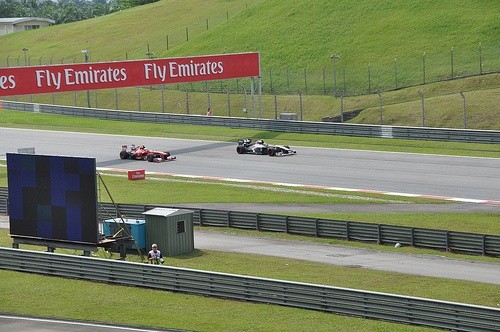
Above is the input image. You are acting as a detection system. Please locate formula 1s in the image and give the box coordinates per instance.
[236,139,296,156]
[119,145,176,163]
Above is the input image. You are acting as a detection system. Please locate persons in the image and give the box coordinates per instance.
[140,145,146,151]
[257,139,265,146]
[147,244,164,265]
[206,108,212,116]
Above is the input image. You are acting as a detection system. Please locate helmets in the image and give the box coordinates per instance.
[140,145,145,149]
[259,139,264,144]
